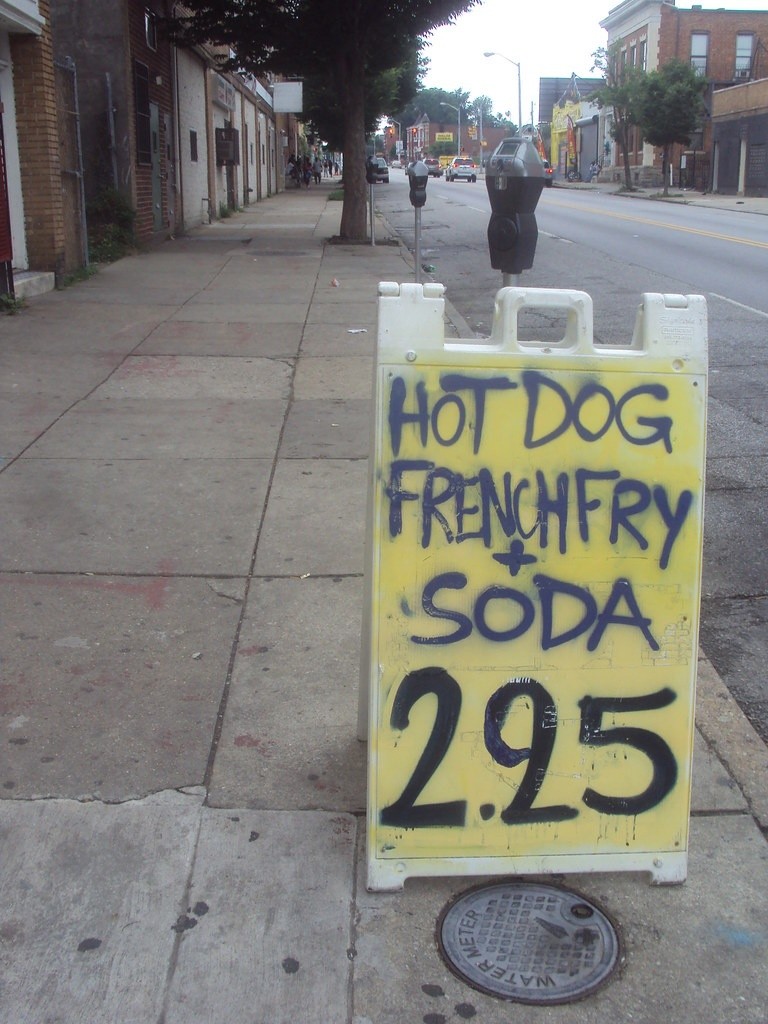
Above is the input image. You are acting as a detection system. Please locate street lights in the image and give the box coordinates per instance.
[387,119,402,161]
[467,101,483,175]
[439,102,461,157]
[483,51,523,136]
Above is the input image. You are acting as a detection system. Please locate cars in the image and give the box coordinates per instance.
[391,160,403,169]
[404,162,414,174]
[543,159,553,188]
[376,157,390,183]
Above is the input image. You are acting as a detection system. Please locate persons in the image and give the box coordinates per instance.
[323,160,339,177]
[586,161,601,182]
[286,154,323,190]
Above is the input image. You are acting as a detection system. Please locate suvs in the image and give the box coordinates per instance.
[422,158,443,177]
[444,157,477,182]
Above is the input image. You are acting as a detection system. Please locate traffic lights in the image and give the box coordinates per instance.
[467,127,473,137]
[472,126,476,135]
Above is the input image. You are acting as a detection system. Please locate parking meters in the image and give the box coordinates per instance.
[365,155,379,246]
[480,136,548,287]
[406,158,431,283]
